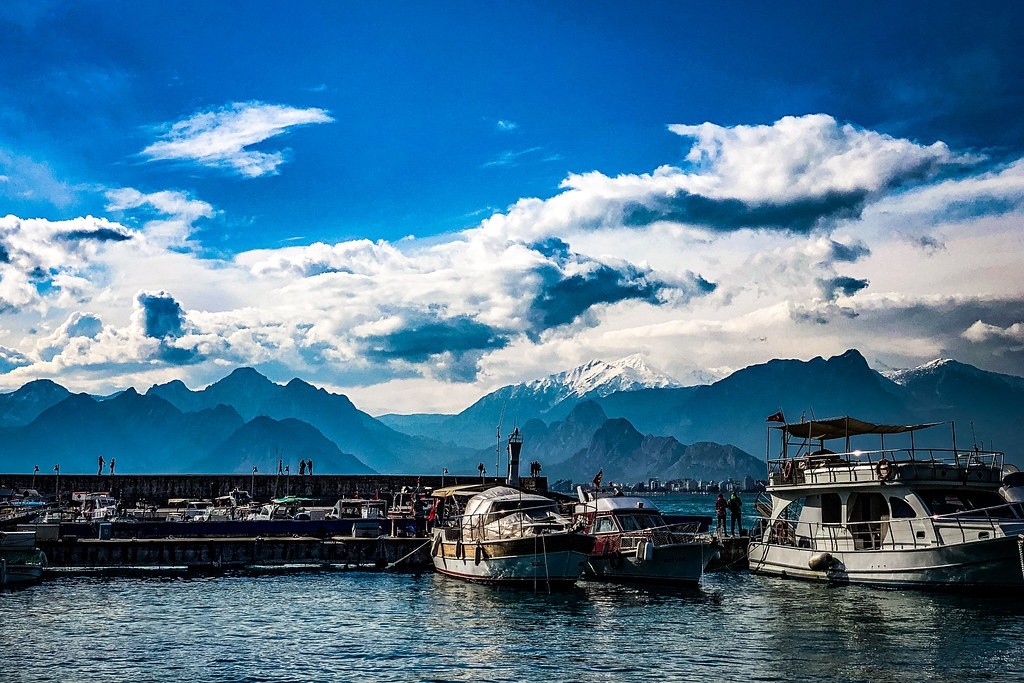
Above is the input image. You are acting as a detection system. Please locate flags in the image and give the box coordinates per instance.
[593,468,603,488]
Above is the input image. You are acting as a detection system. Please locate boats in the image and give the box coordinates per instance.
[0,559,42,589]
[747,405,1024,590]
[0,488,439,522]
[385,427,597,594]
[572,467,720,596]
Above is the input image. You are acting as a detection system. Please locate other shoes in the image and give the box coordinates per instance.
[730,535,735,539]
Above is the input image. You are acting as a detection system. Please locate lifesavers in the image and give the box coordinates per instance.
[784,462,792,480]
[609,550,623,570]
[876,459,893,481]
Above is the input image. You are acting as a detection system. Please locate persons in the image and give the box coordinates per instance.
[411,493,427,538]
[15,552,28,564]
[109,457,116,476]
[433,496,444,528]
[31,547,48,568]
[307,459,313,475]
[727,491,744,538]
[278,459,284,475]
[798,451,810,482]
[97,455,105,475]
[299,459,306,475]
[716,493,730,537]
